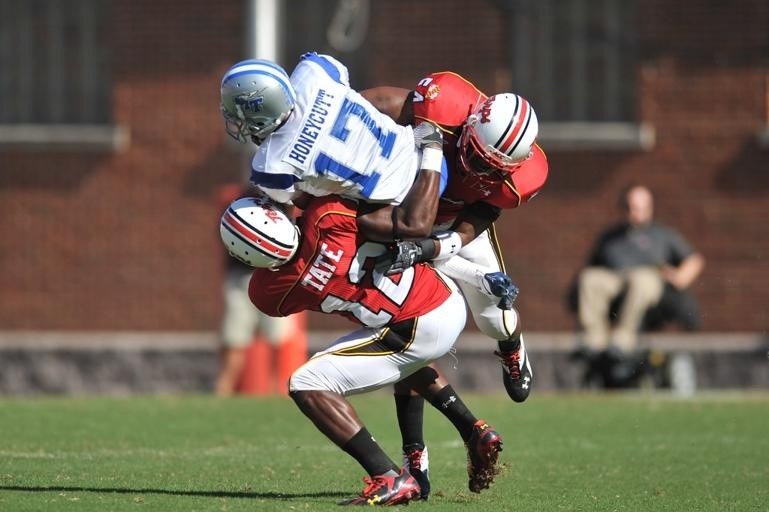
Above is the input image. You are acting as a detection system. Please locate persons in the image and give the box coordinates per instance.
[211,51,548,504]
[565,185,707,363]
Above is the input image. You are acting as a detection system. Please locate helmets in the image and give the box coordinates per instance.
[461,93,539,197]
[220,197,301,272]
[219,58,296,146]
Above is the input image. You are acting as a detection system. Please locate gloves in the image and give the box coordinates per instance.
[386,239,422,274]
[414,122,443,151]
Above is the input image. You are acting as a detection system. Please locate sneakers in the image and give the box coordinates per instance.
[339,467,421,506]
[464,419,503,493]
[494,333,533,402]
[476,268,518,309]
[403,442,430,498]
